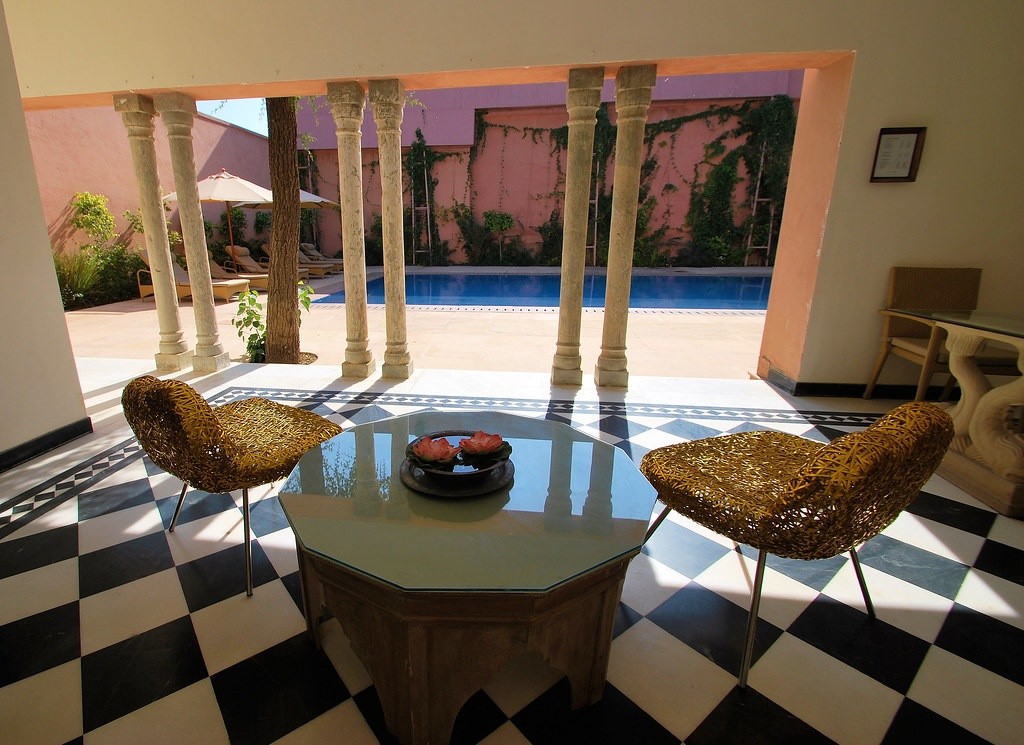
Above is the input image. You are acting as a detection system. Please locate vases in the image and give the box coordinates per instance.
[406,428,508,490]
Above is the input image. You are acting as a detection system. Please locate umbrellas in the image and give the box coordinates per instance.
[233,188,340,208]
[163,168,272,273]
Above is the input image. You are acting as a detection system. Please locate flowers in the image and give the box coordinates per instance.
[405,430,513,472]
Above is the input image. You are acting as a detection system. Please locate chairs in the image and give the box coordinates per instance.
[136,243,343,304]
[120,373,344,597]
[863,267,1024,403]
[640,402,957,707]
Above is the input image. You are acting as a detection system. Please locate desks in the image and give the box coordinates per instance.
[876,308,1024,520]
[278,409,660,745]
